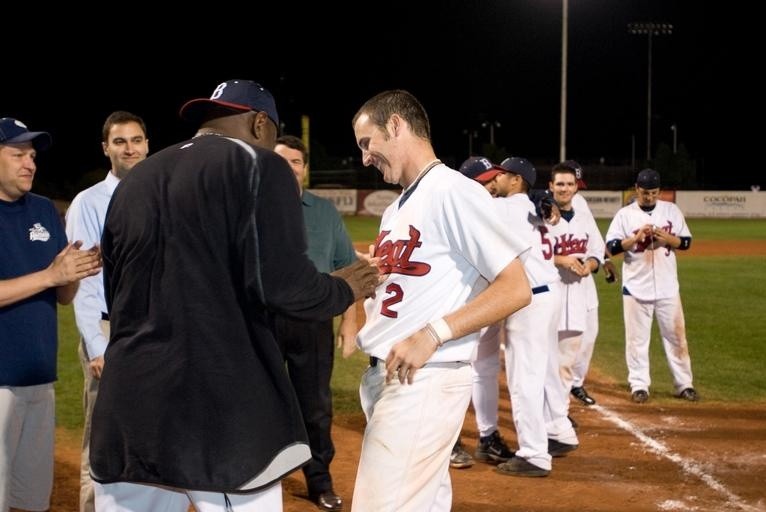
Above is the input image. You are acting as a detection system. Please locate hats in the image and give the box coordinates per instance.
[493,158,538,185]
[179,79,282,130]
[461,156,500,184]
[0,117,51,145]
[637,169,662,190]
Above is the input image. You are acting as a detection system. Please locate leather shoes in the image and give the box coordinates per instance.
[316,491,343,509]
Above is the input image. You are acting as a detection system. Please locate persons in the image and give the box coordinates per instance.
[90,82,379,512]
[351,90,532,512]
[275,136,355,512]
[0,116,104,510]
[65,112,151,512]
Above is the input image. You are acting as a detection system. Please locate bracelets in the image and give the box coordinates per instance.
[428,312,453,346]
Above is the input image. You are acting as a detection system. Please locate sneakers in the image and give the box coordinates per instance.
[496,454,552,478]
[633,389,648,404]
[675,387,698,401]
[549,436,580,457]
[449,444,475,469]
[569,385,598,406]
[476,437,514,464]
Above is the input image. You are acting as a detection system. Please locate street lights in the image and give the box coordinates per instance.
[667,123,679,157]
[623,21,673,159]
[482,114,505,151]
[461,126,478,154]
